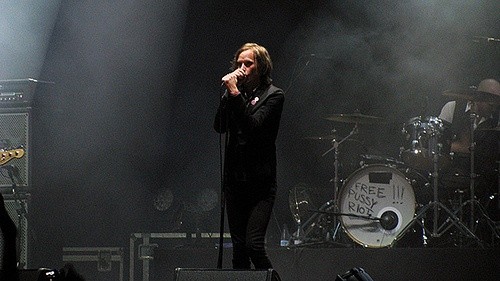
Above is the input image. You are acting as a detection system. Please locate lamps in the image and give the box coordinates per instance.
[153,186,178,212]
[196,188,220,212]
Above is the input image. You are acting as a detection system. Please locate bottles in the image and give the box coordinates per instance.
[279,224,291,247]
[293,224,305,245]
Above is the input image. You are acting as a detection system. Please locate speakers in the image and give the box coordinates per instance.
[0,106,51,271]
[174,268,281,281]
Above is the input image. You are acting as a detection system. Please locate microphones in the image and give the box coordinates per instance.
[471,36,500,46]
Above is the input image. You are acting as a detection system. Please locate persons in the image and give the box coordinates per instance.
[438,79,500,192]
[213,43,285,269]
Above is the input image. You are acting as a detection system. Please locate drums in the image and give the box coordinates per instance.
[336,165,432,249]
[355,153,404,169]
[399,115,456,172]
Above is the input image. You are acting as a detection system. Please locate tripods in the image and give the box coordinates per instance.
[290,141,348,244]
[390,103,500,247]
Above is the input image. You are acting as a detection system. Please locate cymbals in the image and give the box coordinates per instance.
[473,123,500,136]
[439,85,500,103]
[302,133,359,144]
[321,111,385,126]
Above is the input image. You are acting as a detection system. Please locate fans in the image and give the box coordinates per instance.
[288,183,314,246]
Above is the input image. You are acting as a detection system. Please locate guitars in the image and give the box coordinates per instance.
[0,144,26,167]
